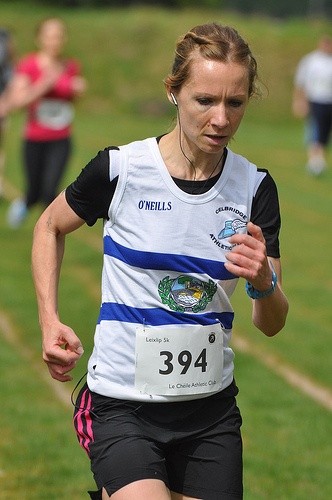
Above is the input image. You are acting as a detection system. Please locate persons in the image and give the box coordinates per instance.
[294,38,332,177]
[31,23,291,500]
[0,18,83,231]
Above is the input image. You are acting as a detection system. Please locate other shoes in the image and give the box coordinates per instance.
[10,200,27,226]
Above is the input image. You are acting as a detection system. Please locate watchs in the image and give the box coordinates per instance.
[244,267,277,299]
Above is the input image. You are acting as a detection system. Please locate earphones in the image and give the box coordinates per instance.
[170,92,178,107]
